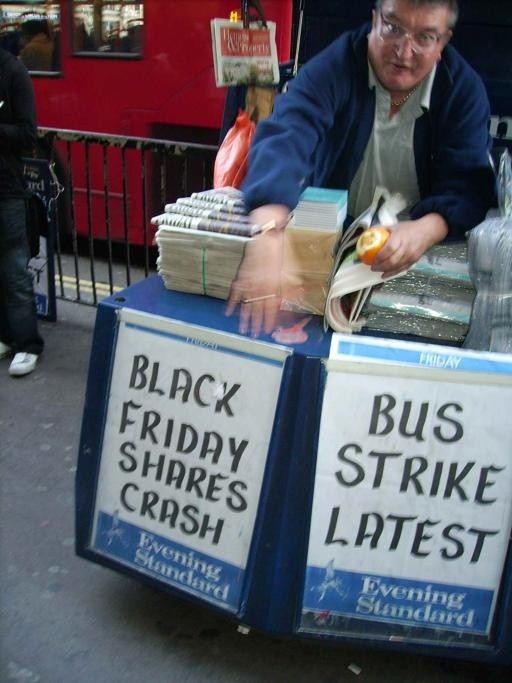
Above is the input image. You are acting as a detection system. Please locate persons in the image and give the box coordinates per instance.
[16,21,53,70]
[223,0,498,337]
[0,47,46,377]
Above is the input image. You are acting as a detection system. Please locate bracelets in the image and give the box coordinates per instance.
[249,218,287,238]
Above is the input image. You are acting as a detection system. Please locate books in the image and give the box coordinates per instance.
[322,183,477,346]
[148,185,349,315]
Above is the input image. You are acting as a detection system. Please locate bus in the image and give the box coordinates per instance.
[1,0,292,247]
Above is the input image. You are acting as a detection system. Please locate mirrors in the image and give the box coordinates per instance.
[0,0,64,76]
[67,2,143,59]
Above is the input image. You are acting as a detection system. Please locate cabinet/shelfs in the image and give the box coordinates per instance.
[75,275,512,669]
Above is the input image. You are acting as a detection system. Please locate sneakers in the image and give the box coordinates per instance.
[1,338,12,360]
[7,351,40,377]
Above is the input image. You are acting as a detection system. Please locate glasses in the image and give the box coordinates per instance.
[379,6,448,48]
